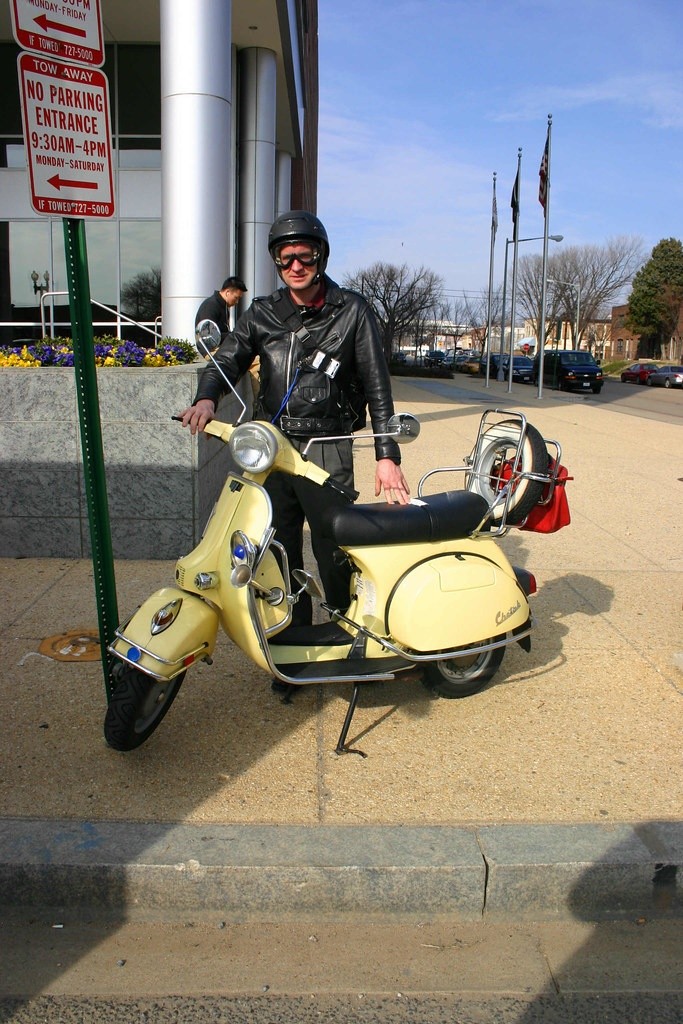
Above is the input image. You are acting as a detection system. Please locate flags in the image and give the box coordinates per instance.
[511,164,520,242]
[492,182,497,244]
[539,127,551,218]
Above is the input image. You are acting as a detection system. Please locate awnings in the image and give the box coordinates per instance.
[518,337,536,347]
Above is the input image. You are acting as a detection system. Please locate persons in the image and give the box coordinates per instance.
[195,276,260,405]
[178,209,410,694]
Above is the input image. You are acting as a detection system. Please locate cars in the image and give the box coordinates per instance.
[424,349,534,385]
[647,365,683,388]
[533,349,605,394]
[621,363,659,385]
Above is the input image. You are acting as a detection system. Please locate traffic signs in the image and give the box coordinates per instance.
[9,0,106,68]
[16,51,116,219]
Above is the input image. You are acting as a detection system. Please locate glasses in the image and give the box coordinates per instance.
[272,240,319,268]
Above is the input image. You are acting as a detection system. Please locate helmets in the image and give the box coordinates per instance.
[267,210,330,274]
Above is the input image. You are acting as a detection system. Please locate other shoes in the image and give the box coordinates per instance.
[272,676,301,693]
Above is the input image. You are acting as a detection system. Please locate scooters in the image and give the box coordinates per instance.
[102,319,575,759]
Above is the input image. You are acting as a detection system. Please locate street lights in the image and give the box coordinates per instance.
[547,280,581,350]
[498,235,563,381]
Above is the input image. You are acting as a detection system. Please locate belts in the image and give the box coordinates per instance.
[253,413,354,433]
